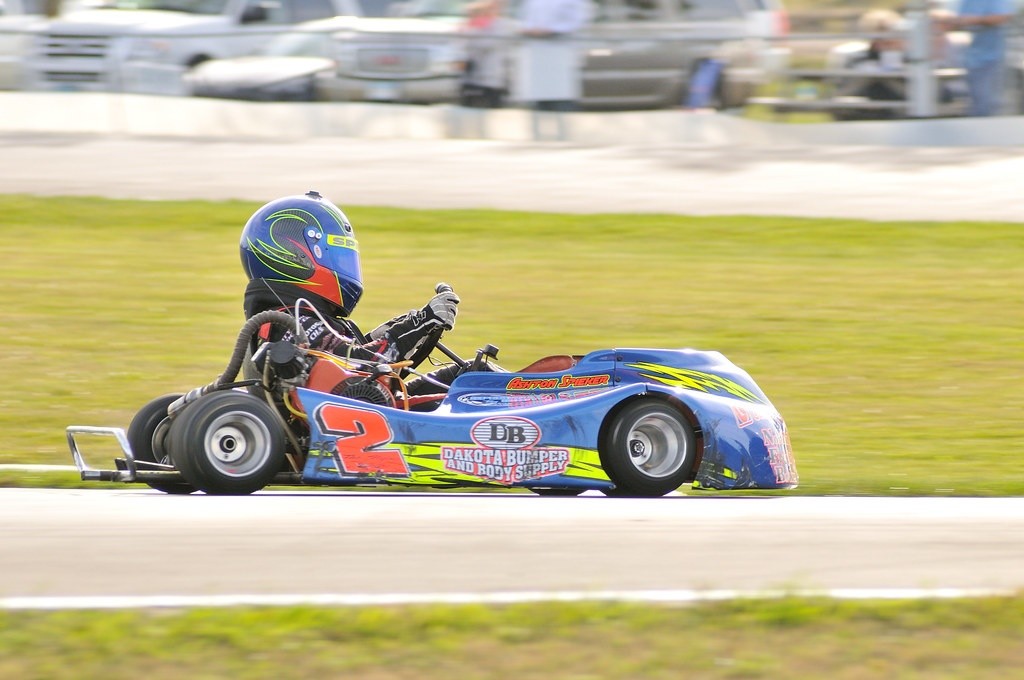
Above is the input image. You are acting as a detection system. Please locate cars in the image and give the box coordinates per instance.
[182,14,374,105]
[312,0,791,117]
[21,0,395,95]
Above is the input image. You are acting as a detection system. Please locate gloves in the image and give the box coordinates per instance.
[418,291,460,330]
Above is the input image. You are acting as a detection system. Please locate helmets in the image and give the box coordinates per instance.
[240,191,363,316]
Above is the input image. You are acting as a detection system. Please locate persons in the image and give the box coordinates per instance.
[239,191,512,412]
[934,0,1017,116]
[450,0,591,112]
[832,9,907,120]
